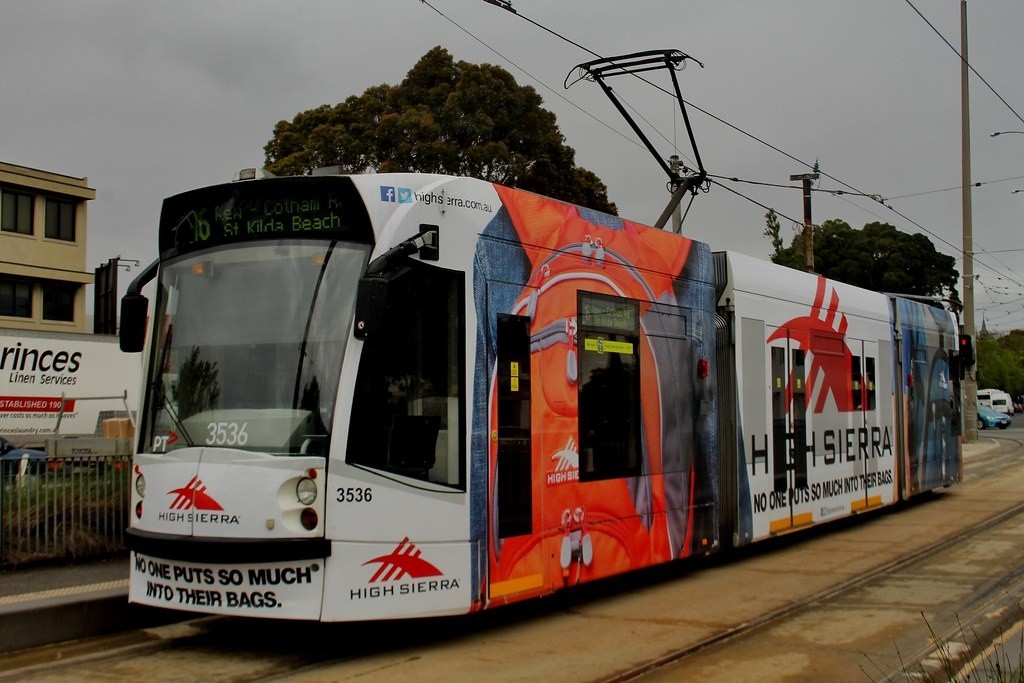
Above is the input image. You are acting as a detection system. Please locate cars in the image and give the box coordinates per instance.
[977,406,1011,430]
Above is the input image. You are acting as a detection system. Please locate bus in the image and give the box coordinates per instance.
[118,48,965,624]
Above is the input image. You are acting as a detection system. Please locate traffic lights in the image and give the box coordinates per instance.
[960,334,972,366]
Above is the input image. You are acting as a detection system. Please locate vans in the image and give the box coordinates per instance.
[977,388,1014,416]
